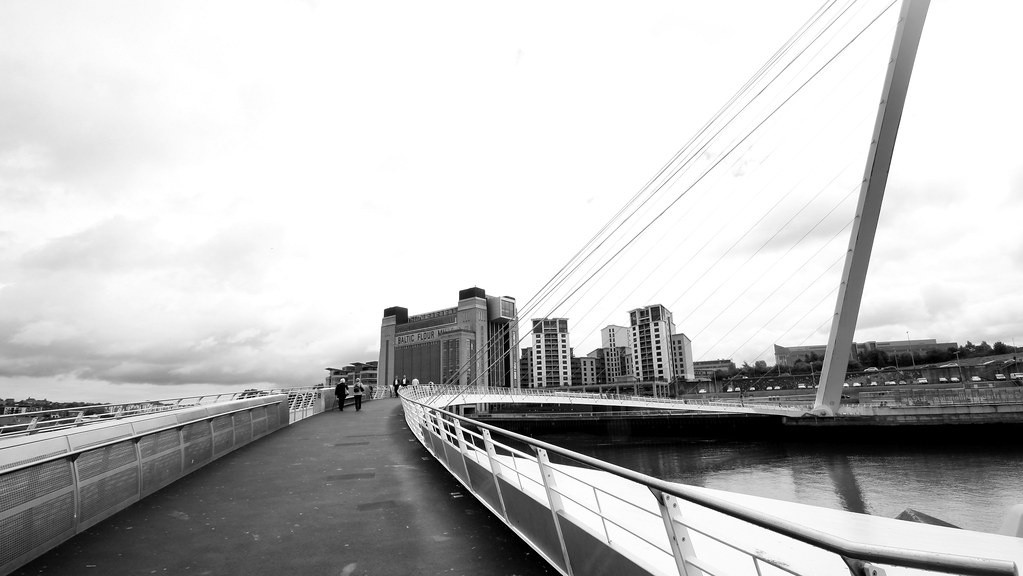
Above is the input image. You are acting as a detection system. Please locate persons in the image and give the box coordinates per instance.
[335,378,348,411]
[684,400,687,405]
[393,376,400,398]
[354,378,365,411]
[412,376,419,395]
[401,374,409,395]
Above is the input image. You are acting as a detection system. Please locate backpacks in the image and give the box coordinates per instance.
[354,384,359,392]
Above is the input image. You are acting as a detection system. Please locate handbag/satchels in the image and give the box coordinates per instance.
[360,383,364,392]
[345,388,350,395]
